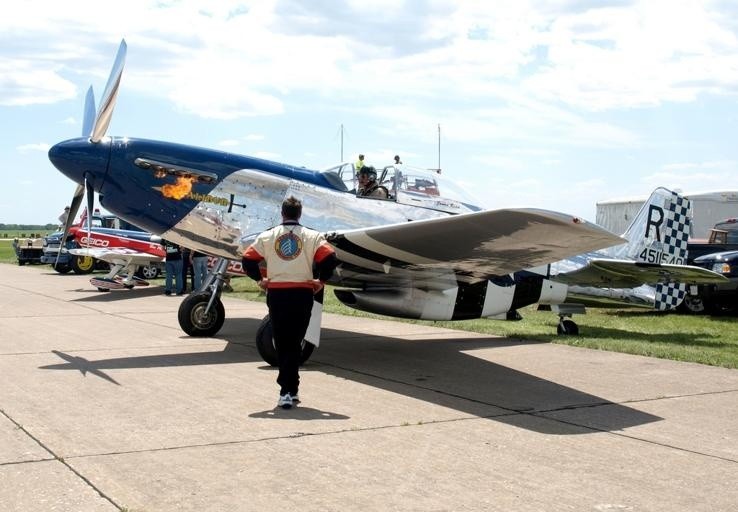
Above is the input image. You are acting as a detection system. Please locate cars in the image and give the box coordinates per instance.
[137,260,193,280]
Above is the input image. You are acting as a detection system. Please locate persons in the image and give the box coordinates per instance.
[357,166,388,199]
[59,207,69,225]
[356,155,365,179]
[242,197,337,409]
[160,238,208,295]
[394,156,402,164]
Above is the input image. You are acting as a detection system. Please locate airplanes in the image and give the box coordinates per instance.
[66,206,271,294]
[46,38,731,339]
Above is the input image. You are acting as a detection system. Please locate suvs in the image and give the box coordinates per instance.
[690,250,738,316]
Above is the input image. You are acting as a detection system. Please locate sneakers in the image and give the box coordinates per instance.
[278,393,299,409]
[166,291,171,295]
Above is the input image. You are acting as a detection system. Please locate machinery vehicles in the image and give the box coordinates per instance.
[677,217,737,314]
[39,209,154,274]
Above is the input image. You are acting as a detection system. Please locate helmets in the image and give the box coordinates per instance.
[360,166,377,182]
